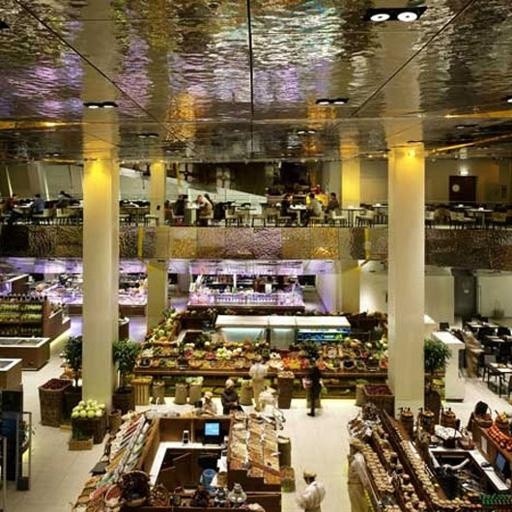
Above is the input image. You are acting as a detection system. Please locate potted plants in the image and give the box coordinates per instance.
[61,335,83,416]
[424,339,453,412]
[112,340,142,411]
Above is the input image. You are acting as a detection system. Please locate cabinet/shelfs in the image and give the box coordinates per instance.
[73,408,510,512]
[133,312,386,398]
[1,293,77,387]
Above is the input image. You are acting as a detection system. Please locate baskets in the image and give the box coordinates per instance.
[68,433,94,451]
[474,402,493,427]
[148,484,170,506]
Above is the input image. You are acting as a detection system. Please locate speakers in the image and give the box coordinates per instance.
[183,430,189,444]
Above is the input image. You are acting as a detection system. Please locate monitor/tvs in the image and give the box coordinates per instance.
[493,451,510,483]
[201,421,224,443]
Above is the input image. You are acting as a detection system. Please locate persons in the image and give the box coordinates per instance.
[2,193,18,222]
[324,192,339,224]
[302,193,322,227]
[201,391,217,413]
[166,194,215,225]
[280,193,296,228]
[54,194,80,209]
[293,470,325,511]
[28,192,46,225]
[211,324,226,343]
[307,364,325,417]
[220,377,244,414]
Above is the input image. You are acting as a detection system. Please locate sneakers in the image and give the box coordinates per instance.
[307,412,314,417]
[254,406,261,412]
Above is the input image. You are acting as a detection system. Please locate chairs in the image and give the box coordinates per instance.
[425,200,511,227]
[34,200,81,223]
[220,199,388,228]
[120,202,181,224]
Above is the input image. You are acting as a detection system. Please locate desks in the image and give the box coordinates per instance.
[441,313,512,400]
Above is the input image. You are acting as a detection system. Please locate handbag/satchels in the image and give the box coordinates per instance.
[230,405,245,413]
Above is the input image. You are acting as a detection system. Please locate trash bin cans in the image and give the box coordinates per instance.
[130,379,150,405]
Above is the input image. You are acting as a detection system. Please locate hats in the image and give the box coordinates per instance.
[303,467,318,478]
[347,436,363,450]
[225,379,234,389]
[204,391,213,400]
